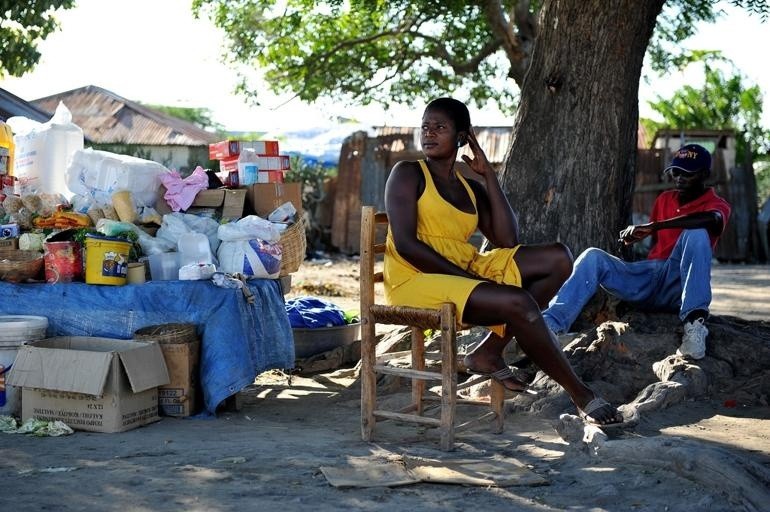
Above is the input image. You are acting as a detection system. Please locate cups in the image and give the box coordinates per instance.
[127,262,145,286]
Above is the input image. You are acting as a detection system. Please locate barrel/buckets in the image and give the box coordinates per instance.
[85,232,133,286]
[179,229,212,266]
[0,314,49,415]
[43,241,81,283]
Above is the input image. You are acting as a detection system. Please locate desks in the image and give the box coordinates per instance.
[0,280,296,420]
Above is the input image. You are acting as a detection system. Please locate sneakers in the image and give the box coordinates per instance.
[676,319,710,360]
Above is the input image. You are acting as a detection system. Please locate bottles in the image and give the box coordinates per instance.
[237,148,259,187]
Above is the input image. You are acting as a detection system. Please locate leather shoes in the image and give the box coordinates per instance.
[663,144,712,175]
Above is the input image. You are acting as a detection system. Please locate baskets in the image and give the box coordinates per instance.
[277,215,308,276]
[132,323,196,344]
[1,249,43,281]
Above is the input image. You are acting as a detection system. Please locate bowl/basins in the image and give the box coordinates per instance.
[292,322,362,359]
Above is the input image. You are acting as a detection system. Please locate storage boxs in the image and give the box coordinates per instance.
[4,335,170,434]
[158,340,201,418]
[156,141,303,221]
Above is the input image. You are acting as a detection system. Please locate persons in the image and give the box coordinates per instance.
[543,144,734,359]
[382,97,625,427]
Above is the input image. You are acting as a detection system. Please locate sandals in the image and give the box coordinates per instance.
[467,365,529,394]
[570,396,624,427]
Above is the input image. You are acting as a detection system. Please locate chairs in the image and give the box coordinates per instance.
[360,205,505,453]
